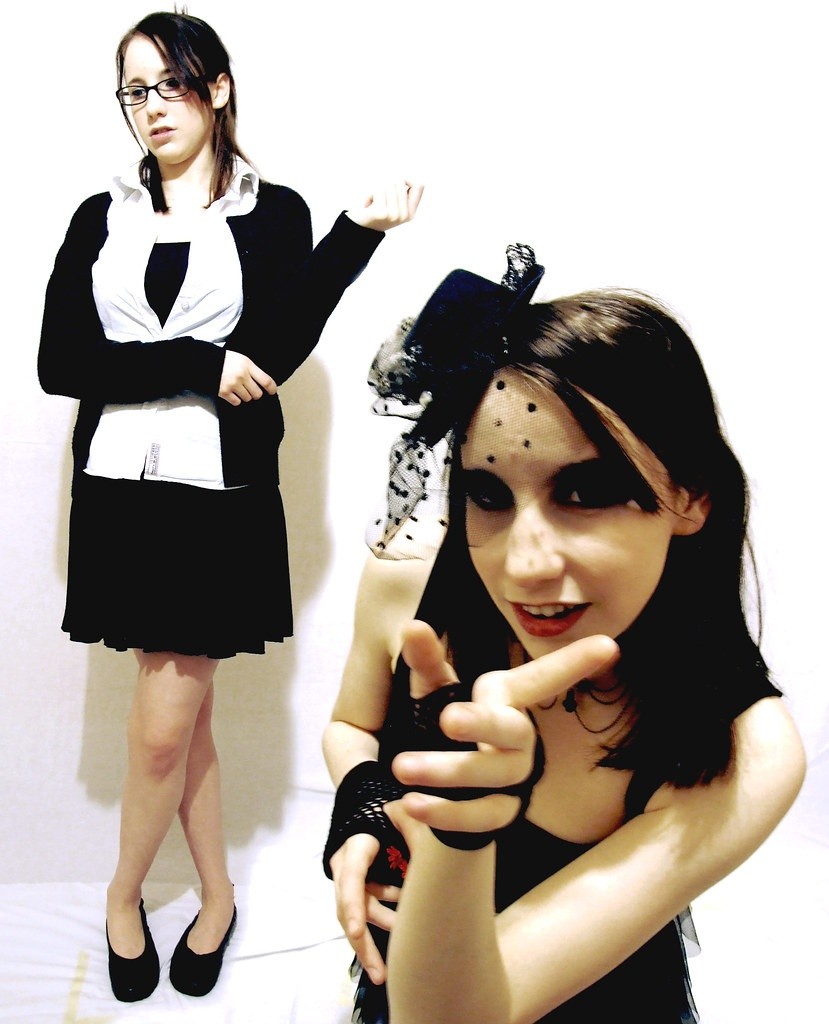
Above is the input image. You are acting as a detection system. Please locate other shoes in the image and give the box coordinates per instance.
[169,904,236,997]
[105,899,160,1003]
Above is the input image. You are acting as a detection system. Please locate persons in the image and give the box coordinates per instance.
[321,237,808,1024]
[35,11,428,1003]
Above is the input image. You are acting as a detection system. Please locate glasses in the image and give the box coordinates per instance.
[117,76,216,106]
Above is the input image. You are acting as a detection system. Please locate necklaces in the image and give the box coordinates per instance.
[562,678,634,734]
